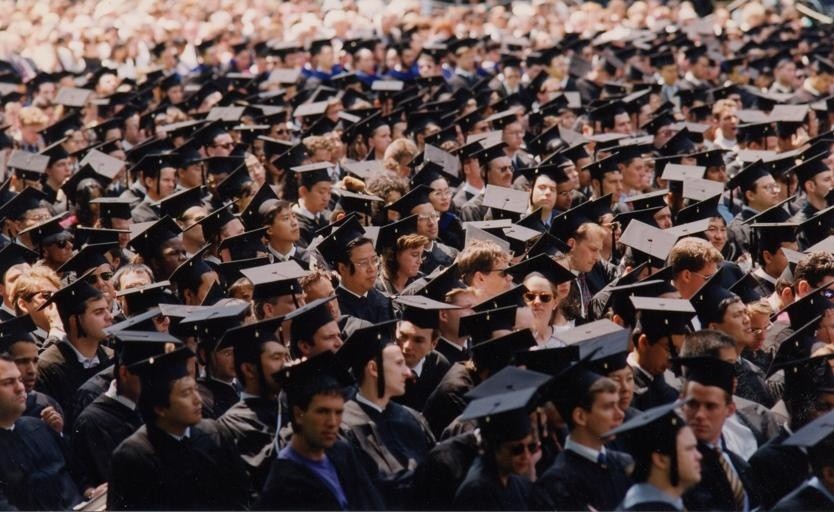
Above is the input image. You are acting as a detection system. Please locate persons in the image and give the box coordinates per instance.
[1,1,834,512]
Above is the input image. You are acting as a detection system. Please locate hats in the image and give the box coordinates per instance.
[0,315,38,341]
[208,106,246,122]
[404,112,436,136]
[539,346,602,403]
[668,355,738,393]
[255,112,290,125]
[172,140,200,156]
[104,92,130,105]
[517,206,546,234]
[738,109,769,140]
[273,142,314,171]
[424,142,460,177]
[294,102,326,123]
[683,175,724,205]
[385,183,434,215]
[604,279,663,315]
[425,101,454,114]
[56,243,113,275]
[635,135,654,154]
[552,201,594,240]
[213,261,260,285]
[464,364,552,399]
[234,125,265,140]
[376,215,417,254]
[6,148,47,180]
[651,156,681,176]
[491,93,521,112]
[423,127,456,144]
[677,192,722,222]
[291,162,332,181]
[78,150,125,178]
[239,261,308,299]
[782,408,834,468]
[604,84,623,95]
[128,347,195,382]
[160,303,204,332]
[503,223,542,257]
[39,115,80,138]
[511,346,580,375]
[502,54,522,67]
[338,190,381,212]
[104,310,162,346]
[258,136,293,157]
[60,169,100,198]
[630,296,696,335]
[359,111,388,128]
[272,349,346,394]
[417,263,462,299]
[456,109,481,131]
[116,281,172,314]
[521,233,569,257]
[623,88,652,111]
[186,202,234,236]
[397,95,423,111]
[673,86,706,105]
[685,44,707,63]
[503,252,575,286]
[765,314,833,395]
[529,123,560,155]
[89,198,140,219]
[449,138,486,155]
[40,137,69,161]
[482,184,530,220]
[810,96,833,119]
[458,387,539,440]
[624,190,668,210]
[337,320,395,369]
[687,149,732,166]
[151,186,203,218]
[549,91,581,109]
[217,163,250,201]
[457,305,517,336]
[410,161,444,187]
[573,329,631,374]
[462,219,512,237]
[797,205,834,242]
[166,119,205,136]
[727,159,767,190]
[804,138,834,159]
[561,142,589,160]
[168,245,212,284]
[650,52,673,68]
[605,58,617,76]
[54,87,91,112]
[94,120,118,133]
[450,87,471,103]
[719,58,743,73]
[728,274,759,303]
[472,78,490,93]
[581,152,619,179]
[108,330,180,363]
[631,84,661,94]
[468,142,508,163]
[640,113,677,135]
[630,64,643,80]
[191,155,243,174]
[240,181,280,230]
[219,228,270,260]
[612,206,661,231]
[815,55,833,75]
[669,122,710,143]
[302,116,335,139]
[73,228,132,242]
[0,187,47,220]
[660,163,707,192]
[765,147,807,172]
[601,399,688,446]
[217,317,283,353]
[752,91,792,109]
[619,219,678,268]
[541,149,568,165]
[770,105,809,124]
[372,76,403,102]
[659,126,695,155]
[705,84,736,97]
[664,218,709,239]
[471,327,537,364]
[130,154,177,170]
[19,211,69,241]
[742,193,796,223]
[0,241,39,284]
[750,223,796,242]
[589,132,627,147]
[603,137,641,162]
[784,151,829,183]
[485,110,517,128]
[315,212,362,238]
[654,100,675,115]
[589,101,628,127]
[394,295,459,328]
[35,268,98,313]
[180,303,248,341]
[316,215,365,265]
[769,282,833,331]
[691,266,742,321]
[124,137,169,156]
[125,220,183,254]
[689,106,712,119]
[593,193,613,215]
[471,284,528,312]
[284,296,337,337]
